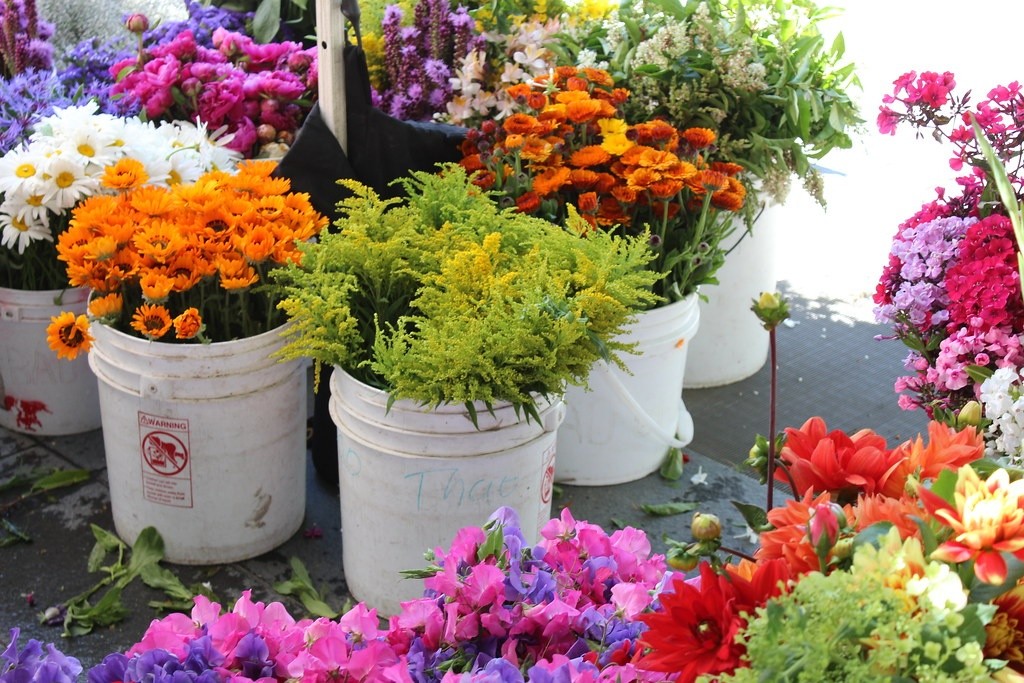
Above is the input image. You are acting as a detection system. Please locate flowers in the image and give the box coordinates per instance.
[0,0,1024,682]
